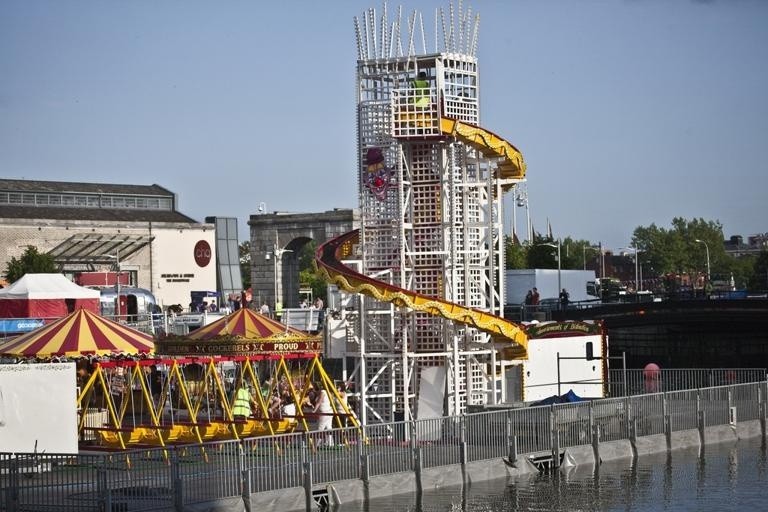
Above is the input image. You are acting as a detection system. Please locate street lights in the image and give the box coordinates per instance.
[696,239,712,281]
[534,236,642,306]
[99,247,123,319]
[265,229,294,311]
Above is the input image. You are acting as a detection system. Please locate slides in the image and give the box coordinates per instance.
[315,108,529,375]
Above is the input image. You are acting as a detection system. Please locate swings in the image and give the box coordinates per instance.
[94,359,303,446]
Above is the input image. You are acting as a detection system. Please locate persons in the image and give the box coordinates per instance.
[558,288,569,312]
[531,286,540,305]
[524,289,532,305]
[81,362,348,452]
[150,295,325,317]
